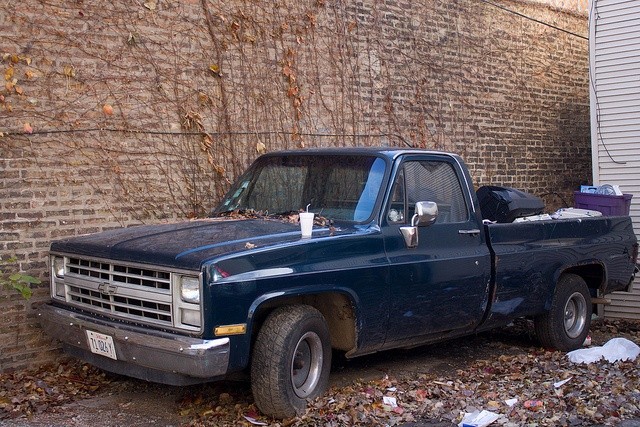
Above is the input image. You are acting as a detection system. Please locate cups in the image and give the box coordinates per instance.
[298,213,315,237]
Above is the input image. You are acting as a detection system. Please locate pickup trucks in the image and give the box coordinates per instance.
[36,146,639,419]
